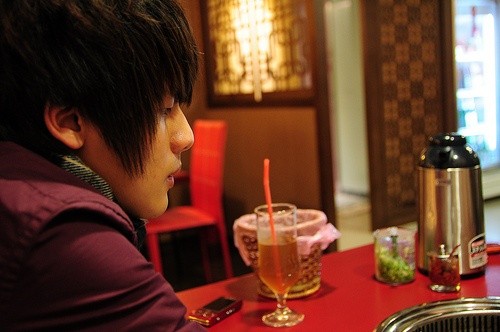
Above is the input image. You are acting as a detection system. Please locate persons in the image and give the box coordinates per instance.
[0,0,204,332]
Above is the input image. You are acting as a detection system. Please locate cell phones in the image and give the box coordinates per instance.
[186,297,242,325]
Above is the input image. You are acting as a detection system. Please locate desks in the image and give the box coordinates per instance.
[176,242,500,332]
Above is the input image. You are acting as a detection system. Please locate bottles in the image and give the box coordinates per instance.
[426,243,461,292]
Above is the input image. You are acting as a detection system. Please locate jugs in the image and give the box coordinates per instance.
[415,132,485,281]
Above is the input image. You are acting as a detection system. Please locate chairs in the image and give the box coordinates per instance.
[141,119,233,279]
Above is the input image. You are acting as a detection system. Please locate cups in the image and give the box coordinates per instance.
[372,226,416,285]
[238,209,328,299]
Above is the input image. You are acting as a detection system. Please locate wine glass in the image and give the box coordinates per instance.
[255,203,305,327]
